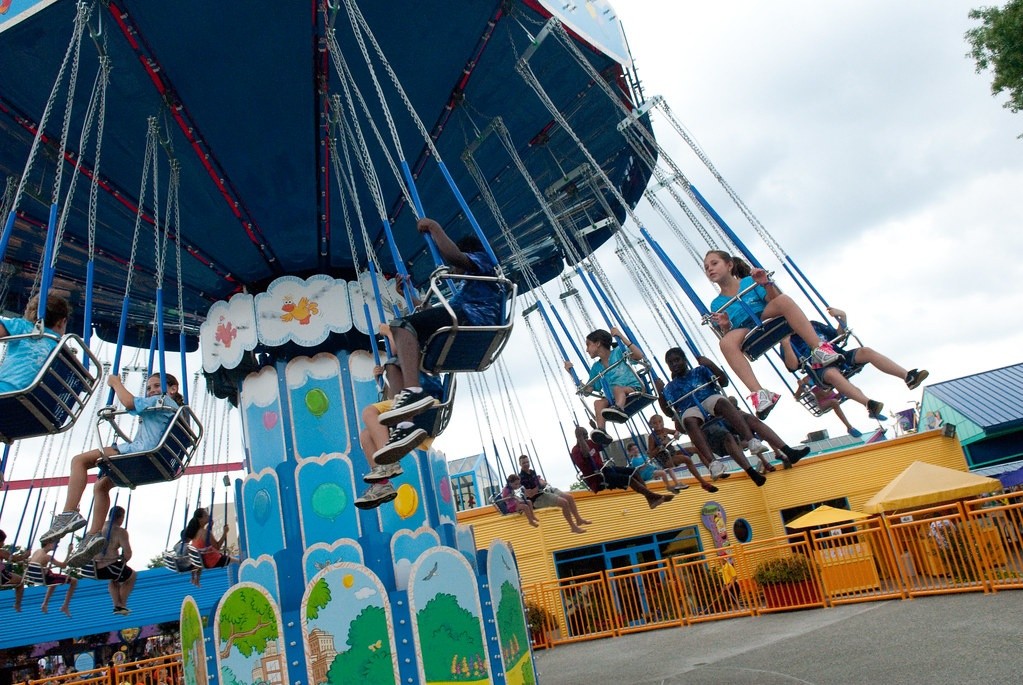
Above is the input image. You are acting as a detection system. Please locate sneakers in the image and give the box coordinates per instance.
[354,480,397,509]
[811,342,840,370]
[867,398,884,416]
[905,368,929,390]
[363,460,403,483]
[746,387,781,420]
[67,530,107,568]
[709,460,726,480]
[372,424,427,465]
[747,438,769,455]
[378,388,434,425]
[39,508,87,542]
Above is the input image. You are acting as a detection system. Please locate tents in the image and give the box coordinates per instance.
[864,460,1005,585]
[1000,466,1023,504]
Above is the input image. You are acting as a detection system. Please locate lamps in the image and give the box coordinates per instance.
[942,423,956,438]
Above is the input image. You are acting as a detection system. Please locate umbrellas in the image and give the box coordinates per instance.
[784,502,872,547]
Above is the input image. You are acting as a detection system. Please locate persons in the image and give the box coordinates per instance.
[24,638,184,685]
[40,374,184,567]
[354,218,503,510]
[184,508,238,568]
[648,415,730,492]
[519,455,592,533]
[502,474,539,529]
[94,506,135,615]
[30,538,77,618]
[565,327,646,444]
[572,419,693,509]
[0,290,68,487]
[173,529,200,588]
[704,251,847,421]
[655,348,811,488]
[780,307,930,437]
[920,512,956,547]
[0,529,32,612]
[721,559,739,611]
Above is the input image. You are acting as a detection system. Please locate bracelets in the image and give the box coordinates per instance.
[729,321,734,330]
[628,342,633,347]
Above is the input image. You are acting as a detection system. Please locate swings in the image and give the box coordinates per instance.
[92,99,206,491]
[320,0,522,442]
[452,3,869,518]
[0,332,232,589]
[0,3,114,442]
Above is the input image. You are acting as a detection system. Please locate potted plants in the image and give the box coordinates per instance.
[525,603,560,647]
[751,554,827,609]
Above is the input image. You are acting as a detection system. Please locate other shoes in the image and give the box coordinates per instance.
[113,606,132,614]
[600,405,628,423]
[591,429,613,445]
[868,412,888,421]
[647,447,809,511]
[847,427,862,437]
